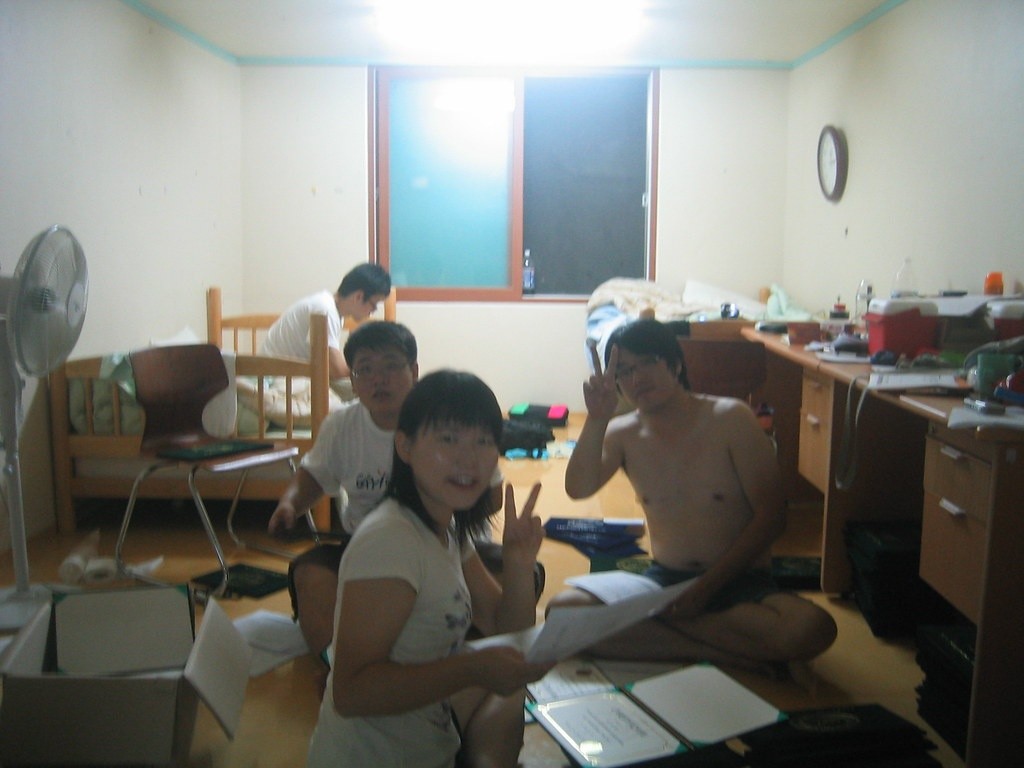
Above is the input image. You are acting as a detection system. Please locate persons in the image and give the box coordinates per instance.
[258,264,391,410]
[305,370,543,768]
[544,317,837,701]
[269,322,544,694]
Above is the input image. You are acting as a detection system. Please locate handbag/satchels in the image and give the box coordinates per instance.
[506,403,571,427]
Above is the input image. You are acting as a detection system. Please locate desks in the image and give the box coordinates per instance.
[740,327,1024,768]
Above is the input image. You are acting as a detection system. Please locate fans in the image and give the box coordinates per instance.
[0,224,90,630]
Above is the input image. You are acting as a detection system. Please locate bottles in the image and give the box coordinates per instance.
[523,249,535,292]
[897,255,916,291]
[856,278,869,318]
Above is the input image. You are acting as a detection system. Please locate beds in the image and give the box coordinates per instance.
[48,285,397,535]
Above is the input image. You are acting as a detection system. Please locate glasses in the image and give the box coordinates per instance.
[368,300,378,311]
[616,357,658,382]
[349,358,409,383]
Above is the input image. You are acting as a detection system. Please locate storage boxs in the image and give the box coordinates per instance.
[0,583,250,768]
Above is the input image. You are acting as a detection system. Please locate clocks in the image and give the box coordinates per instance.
[817,124,846,200]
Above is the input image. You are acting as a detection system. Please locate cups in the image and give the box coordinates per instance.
[977,353,1014,404]
[984,271,1003,295]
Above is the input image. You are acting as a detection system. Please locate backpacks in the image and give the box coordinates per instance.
[501,419,555,460]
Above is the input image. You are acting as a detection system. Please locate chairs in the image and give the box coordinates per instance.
[116,343,322,600]
[675,336,776,561]
